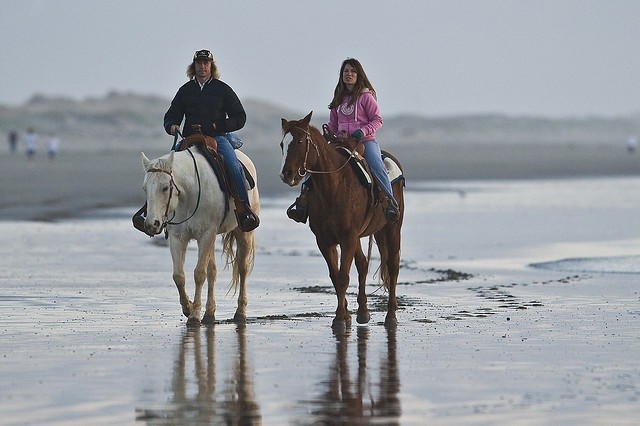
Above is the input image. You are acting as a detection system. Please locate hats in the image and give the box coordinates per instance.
[192,49,213,59]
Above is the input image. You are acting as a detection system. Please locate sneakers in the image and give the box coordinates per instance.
[385,206,397,219]
[239,213,256,230]
[133,215,155,237]
[289,206,306,223]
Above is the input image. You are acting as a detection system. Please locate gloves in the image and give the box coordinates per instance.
[324,132,333,141]
[353,128,363,140]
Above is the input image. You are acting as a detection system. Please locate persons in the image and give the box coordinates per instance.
[25,128,40,163]
[45,133,60,170]
[132,49,260,238]
[287,58,401,224]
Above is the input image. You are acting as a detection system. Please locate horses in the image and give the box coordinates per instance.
[139,143,261,327]
[278,110,405,335]
